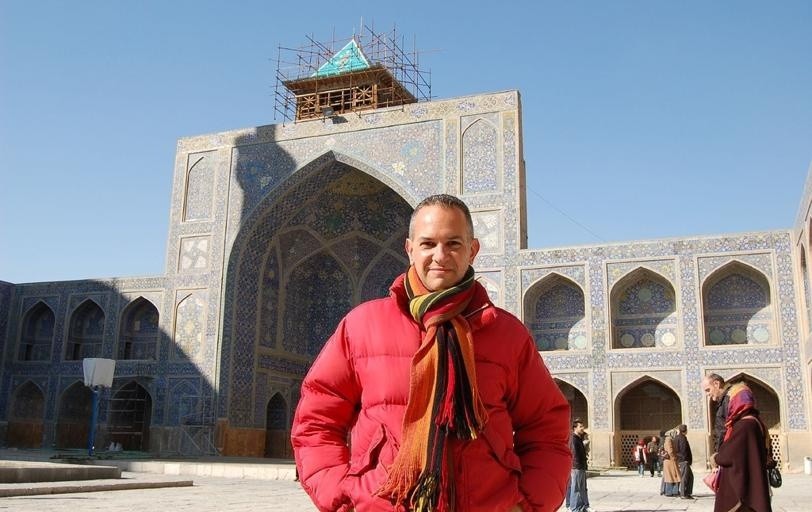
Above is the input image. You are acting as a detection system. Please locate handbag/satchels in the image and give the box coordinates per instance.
[770,468,782,488]
[657,447,671,460]
[703,468,719,491]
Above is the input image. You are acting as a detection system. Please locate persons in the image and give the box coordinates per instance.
[291,194,571,512]
[631,425,695,499]
[566,417,589,512]
[703,373,772,512]
